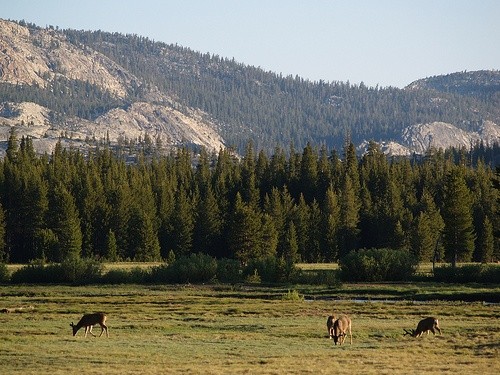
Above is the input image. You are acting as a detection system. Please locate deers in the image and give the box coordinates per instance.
[402,317,442,339]
[327,315,352,346]
[70,312,109,337]
